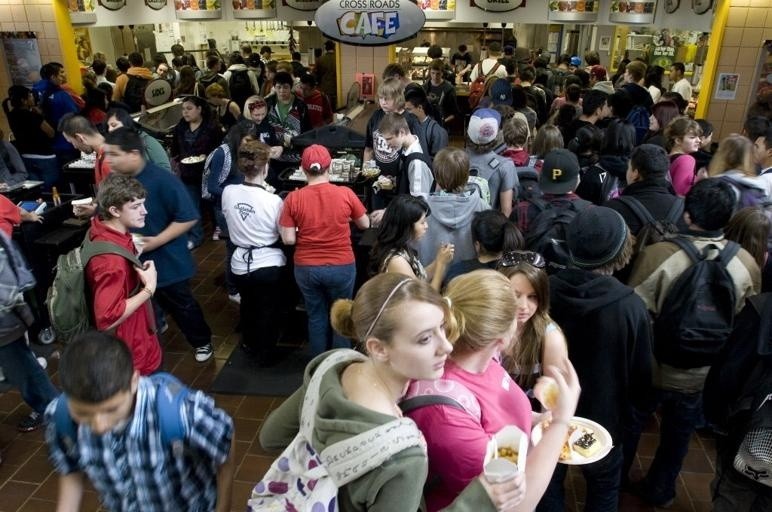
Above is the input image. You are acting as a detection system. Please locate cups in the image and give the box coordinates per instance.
[494,251,546,271]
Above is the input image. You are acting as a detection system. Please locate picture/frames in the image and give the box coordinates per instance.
[338,103,381,137]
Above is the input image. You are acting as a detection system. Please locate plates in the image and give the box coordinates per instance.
[410,61,430,79]
[610,26,697,76]
[14,191,89,320]
[273,166,374,212]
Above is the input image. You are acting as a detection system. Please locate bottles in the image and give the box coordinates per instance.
[301,143,331,172]
[625,61,647,77]
[466,108,501,145]
[538,147,580,195]
[584,50,600,66]
[504,45,513,55]
[570,56,581,65]
[488,41,501,51]
[490,78,514,107]
[262,46,275,54]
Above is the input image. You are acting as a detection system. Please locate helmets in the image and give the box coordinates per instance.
[180,156,204,165]
[531,417,613,466]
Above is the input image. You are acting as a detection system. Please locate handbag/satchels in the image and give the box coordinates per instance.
[143,287,153,298]
[547,415,572,428]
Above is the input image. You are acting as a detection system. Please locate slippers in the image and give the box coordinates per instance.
[247,348,428,512]
[468,61,501,109]
[551,70,571,97]
[721,175,771,212]
[565,206,627,270]
[613,194,686,257]
[46,226,143,352]
[617,87,650,142]
[31,84,65,124]
[228,69,252,104]
[524,198,593,277]
[0,231,37,313]
[514,156,541,202]
[201,143,232,199]
[461,155,513,204]
[717,292,772,496]
[124,73,153,115]
[656,236,741,371]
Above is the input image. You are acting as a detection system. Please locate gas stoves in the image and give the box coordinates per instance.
[379,175,392,190]
[71,196,94,217]
[572,431,598,459]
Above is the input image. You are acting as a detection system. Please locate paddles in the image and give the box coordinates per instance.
[210,339,307,396]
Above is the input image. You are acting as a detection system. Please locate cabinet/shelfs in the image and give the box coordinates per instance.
[52,186,61,207]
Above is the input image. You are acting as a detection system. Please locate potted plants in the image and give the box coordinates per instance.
[470,0,527,13]
[691,0,712,14]
[282,1,323,12]
[664,1,680,14]
[98,0,126,10]
[145,0,167,10]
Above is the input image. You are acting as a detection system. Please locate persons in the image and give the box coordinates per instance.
[113,56,130,84]
[504,63,517,82]
[377,113,437,198]
[2,84,61,193]
[574,69,590,98]
[404,86,449,161]
[382,63,435,116]
[753,130,772,197]
[549,205,655,512]
[500,117,544,173]
[449,44,472,79]
[206,39,226,73]
[223,51,260,114]
[222,95,269,146]
[291,51,304,77]
[259,60,278,99]
[404,145,493,275]
[42,331,235,512]
[739,114,770,143]
[511,84,538,132]
[544,53,576,81]
[532,122,565,159]
[603,143,689,288]
[108,109,172,173]
[92,60,117,90]
[156,62,170,77]
[0,130,29,189]
[0,194,45,239]
[644,100,679,147]
[597,118,636,188]
[622,50,631,64]
[669,62,692,116]
[517,66,547,128]
[723,206,772,268]
[755,87,772,107]
[553,103,577,148]
[150,53,180,89]
[83,52,117,83]
[596,93,634,134]
[570,90,610,139]
[748,101,771,122]
[612,60,654,118]
[643,65,664,104]
[440,210,525,296]
[198,55,231,98]
[259,71,313,184]
[98,84,133,114]
[172,55,188,72]
[569,56,581,73]
[295,74,334,129]
[241,44,265,87]
[533,57,553,87]
[221,140,297,367]
[567,124,620,206]
[541,51,552,71]
[81,87,110,130]
[663,116,704,195]
[427,86,441,105]
[172,45,200,73]
[398,268,581,512]
[610,59,631,86]
[478,73,499,101]
[276,61,295,75]
[549,74,583,115]
[707,133,757,215]
[422,59,461,130]
[257,272,524,512]
[709,251,772,512]
[564,84,584,120]
[366,194,456,294]
[111,52,155,114]
[493,104,513,129]
[490,77,531,138]
[172,65,205,98]
[427,44,452,81]
[469,40,507,81]
[621,178,763,506]
[589,65,615,94]
[0,227,61,432]
[694,119,715,174]
[205,82,242,131]
[49,62,88,112]
[186,54,204,81]
[313,41,336,110]
[500,45,518,66]
[32,63,81,161]
[533,73,553,112]
[57,112,112,220]
[508,148,594,251]
[462,107,520,218]
[260,45,275,64]
[278,143,370,360]
[362,76,432,211]
[83,172,163,375]
[657,92,688,114]
[693,36,708,65]
[583,49,601,74]
[104,127,213,362]
[496,249,569,512]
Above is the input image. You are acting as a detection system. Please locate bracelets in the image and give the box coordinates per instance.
[72,198,92,215]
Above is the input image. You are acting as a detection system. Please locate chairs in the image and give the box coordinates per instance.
[212,226,222,241]
[16,410,50,433]
[194,343,213,362]
[36,357,48,369]
[227,293,241,306]
